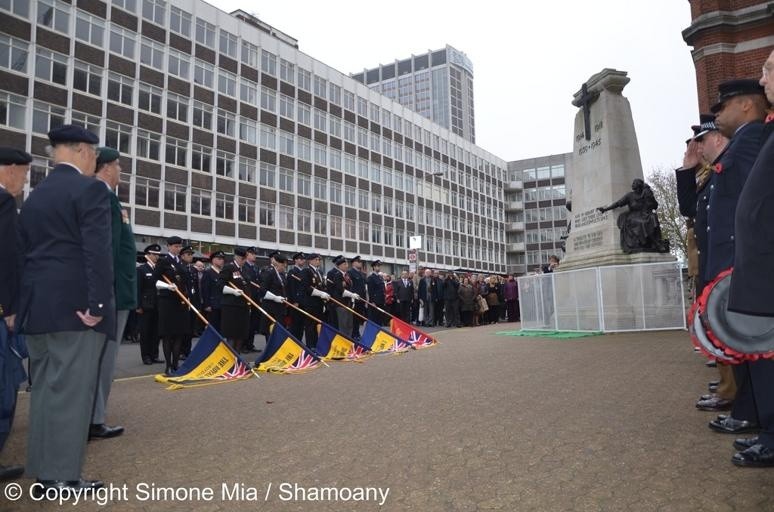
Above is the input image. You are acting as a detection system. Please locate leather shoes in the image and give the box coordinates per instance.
[0,462,24,480]
[251,347,261,352]
[696,380,774,466]
[88,424,123,440]
[241,348,249,353]
[144,353,192,376]
[34,475,103,499]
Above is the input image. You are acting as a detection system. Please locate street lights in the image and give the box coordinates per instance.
[412,172,443,274]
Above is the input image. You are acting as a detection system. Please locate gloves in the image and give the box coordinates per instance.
[310,288,330,304]
[222,285,244,297]
[154,279,177,292]
[342,289,360,302]
[263,290,286,304]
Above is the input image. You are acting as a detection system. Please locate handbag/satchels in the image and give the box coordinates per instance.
[477,295,489,312]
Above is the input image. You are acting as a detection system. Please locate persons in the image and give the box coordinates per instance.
[124,237,559,373]
[0,145,33,481]
[14,124,118,500]
[597,178,661,253]
[89,147,138,440]
[675,50,773,468]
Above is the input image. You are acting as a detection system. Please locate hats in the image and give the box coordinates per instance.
[144,236,195,256]
[332,255,361,266]
[47,125,99,144]
[710,79,765,113]
[95,147,119,164]
[209,247,257,259]
[371,260,382,266]
[694,114,718,141]
[0,146,32,166]
[268,249,320,263]
[691,126,700,133]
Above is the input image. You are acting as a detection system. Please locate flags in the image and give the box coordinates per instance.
[154,323,253,388]
